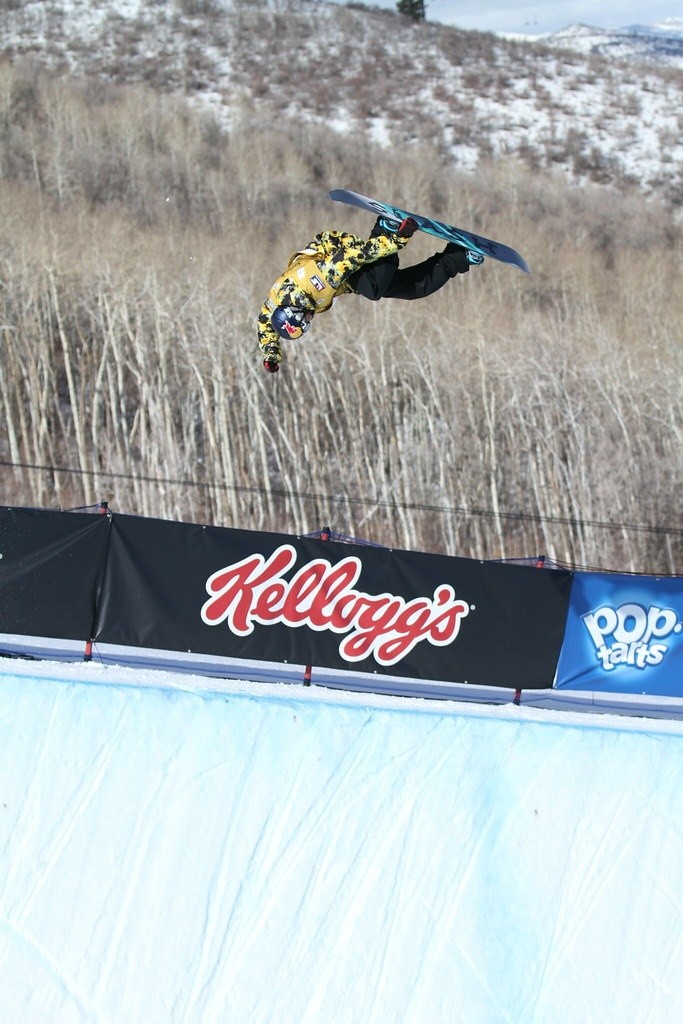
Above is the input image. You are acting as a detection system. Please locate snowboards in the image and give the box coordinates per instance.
[326,188,535,277]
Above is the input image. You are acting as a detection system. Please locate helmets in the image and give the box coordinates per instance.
[271,308,303,340]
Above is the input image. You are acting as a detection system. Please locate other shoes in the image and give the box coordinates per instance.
[381,218,401,232]
[466,250,484,266]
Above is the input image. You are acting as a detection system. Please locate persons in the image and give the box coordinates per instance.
[258,215,484,373]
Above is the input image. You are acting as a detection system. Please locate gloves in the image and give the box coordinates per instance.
[264,360,280,373]
[398,217,418,237]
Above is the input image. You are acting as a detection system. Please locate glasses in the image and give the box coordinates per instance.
[299,321,310,333]
